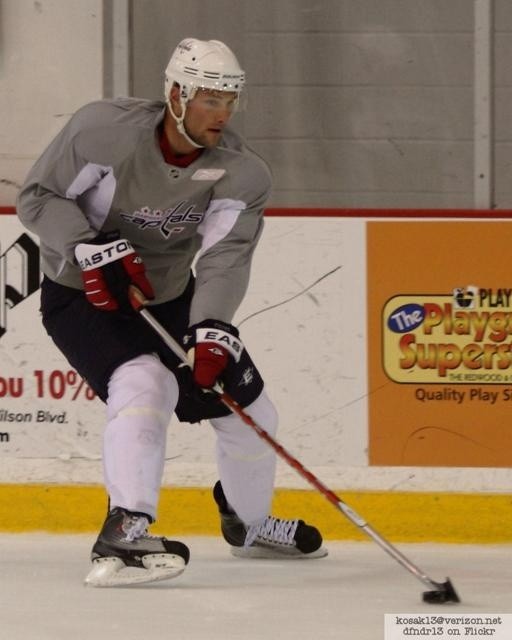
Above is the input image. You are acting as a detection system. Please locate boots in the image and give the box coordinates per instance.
[213,482,323,554]
[91,495,191,568]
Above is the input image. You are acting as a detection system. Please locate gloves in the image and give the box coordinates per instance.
[73,232,156,315]
[179,317,244,395]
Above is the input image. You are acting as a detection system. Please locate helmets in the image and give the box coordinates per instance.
[165,38,246,104]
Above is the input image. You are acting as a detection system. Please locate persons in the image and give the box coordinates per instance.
[15,36,323,569]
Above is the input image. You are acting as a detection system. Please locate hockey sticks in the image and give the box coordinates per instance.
[130,286,458,606]
